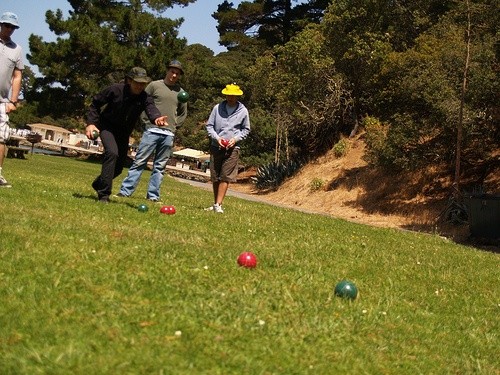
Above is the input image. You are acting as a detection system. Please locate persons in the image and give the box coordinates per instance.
[204,84,251,215]
[116,60,188,203]
[85,66,169,203]
[0,12,26,184]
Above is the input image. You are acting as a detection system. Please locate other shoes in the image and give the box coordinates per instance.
[98,195,110,203]
[0,175,12,188]
[154,198,163,204]
[112,192,129,198]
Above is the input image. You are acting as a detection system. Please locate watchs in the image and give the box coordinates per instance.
[10,101,18,107]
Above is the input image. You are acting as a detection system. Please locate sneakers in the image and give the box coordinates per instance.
[213,203,223,214]
[204,206,214,211]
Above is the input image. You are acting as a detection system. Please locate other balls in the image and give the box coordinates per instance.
[90,130,99,140]
[334,282,358,301]
[178,91,190,102]
[138,204,149,212]
[221,139,228,146]
[237,252,257,268]
[160,206,176,214]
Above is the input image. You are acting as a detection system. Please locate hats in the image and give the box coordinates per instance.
[0,12,20,29]
[222,84,243,96]
[166,60,185,76]
[126,66,151,83]
